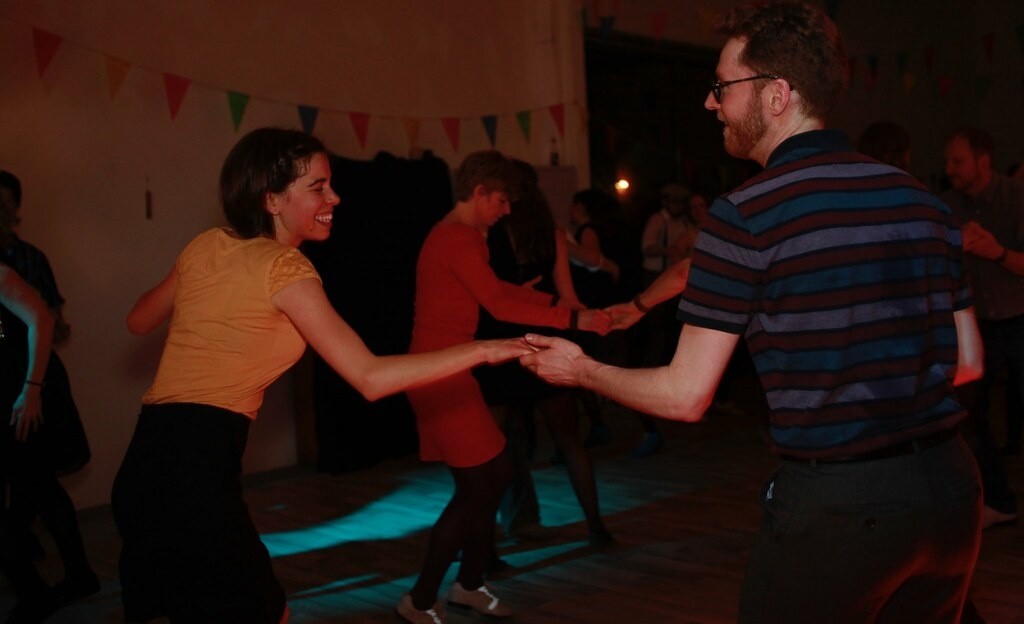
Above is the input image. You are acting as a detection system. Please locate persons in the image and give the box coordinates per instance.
[84,124,543,624]
[939,119,1024,529]
[859,119,990,255]
[0,167,89,623]
[519,6,986,624]
[375,148,714,624]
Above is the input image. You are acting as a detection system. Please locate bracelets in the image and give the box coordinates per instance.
[633,295,649,312]
[994,248,1008,264]
[570,308,579,330]
[550,296,560,307]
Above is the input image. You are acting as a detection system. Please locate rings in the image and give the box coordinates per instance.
[968,238,973,243]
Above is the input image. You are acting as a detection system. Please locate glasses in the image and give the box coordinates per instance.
[713,75,794,104]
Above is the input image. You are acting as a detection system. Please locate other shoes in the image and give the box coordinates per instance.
[396,592,449,624]
[509,523,564,546]
[982,502,1016,533]
[586,533,627,556]
[445,581,513,621]
